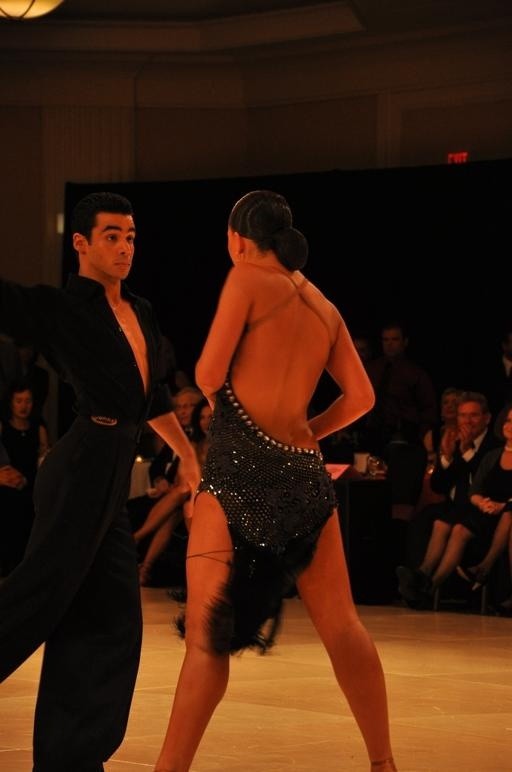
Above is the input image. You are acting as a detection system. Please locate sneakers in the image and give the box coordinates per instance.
[396,564,441,609]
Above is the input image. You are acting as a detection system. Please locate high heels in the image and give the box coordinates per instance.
[456,563,483,591]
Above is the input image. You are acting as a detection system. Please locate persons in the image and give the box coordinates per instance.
[154,188,396,771]
[0,191,200,771]
[0,323,511,604]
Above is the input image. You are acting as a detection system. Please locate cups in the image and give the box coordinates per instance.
[368,455,381,478]
